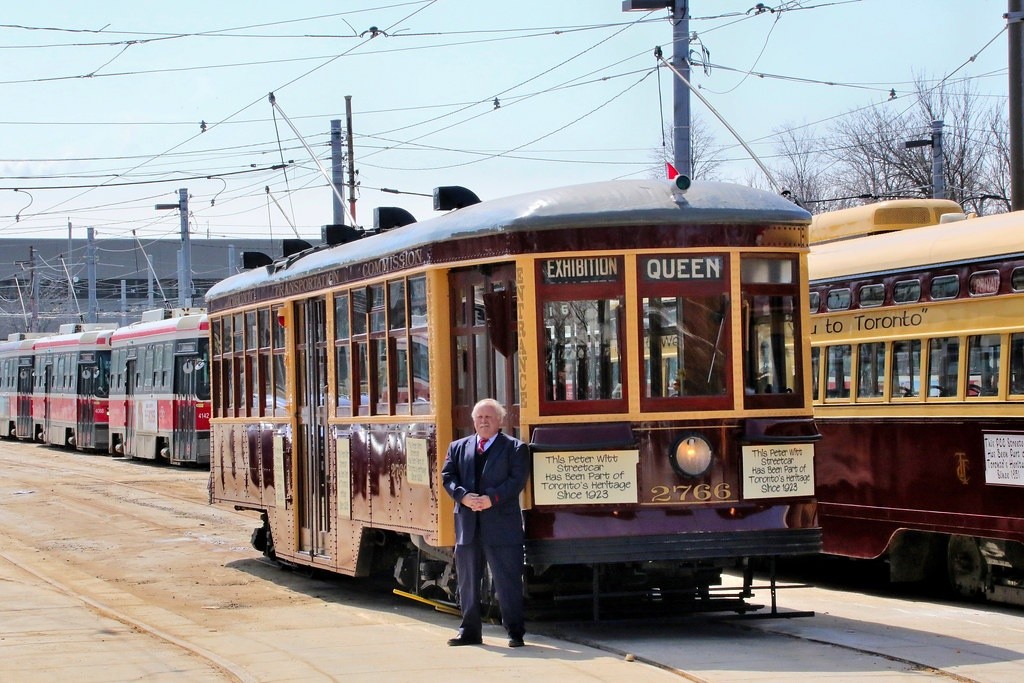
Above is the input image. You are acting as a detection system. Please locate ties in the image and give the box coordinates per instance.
[477,438,489,455]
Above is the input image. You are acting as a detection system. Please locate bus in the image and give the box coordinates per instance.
[108,232,209,467]
[609,199,1023,604]
[0,275,54,441]
[35,255,113,452]
[207,48,824,633]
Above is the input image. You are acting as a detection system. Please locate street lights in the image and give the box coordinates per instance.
[898,118,948,203]
[155,187,193,308]
[619,0,694,176]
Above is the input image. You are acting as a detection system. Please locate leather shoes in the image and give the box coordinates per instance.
[509,638,524,647]
[447,634,482,646]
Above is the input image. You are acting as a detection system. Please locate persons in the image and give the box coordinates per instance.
[441,398,532,647]
